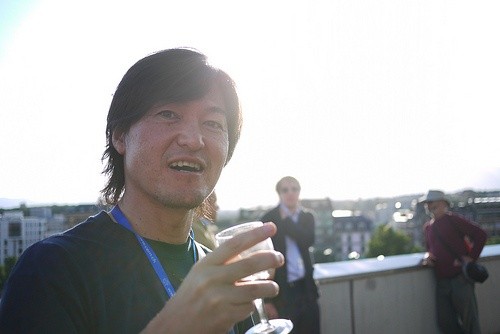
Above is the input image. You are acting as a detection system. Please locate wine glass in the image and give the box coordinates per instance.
[215,220,294,334]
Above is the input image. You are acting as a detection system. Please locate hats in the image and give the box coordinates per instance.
[414,189,453,212]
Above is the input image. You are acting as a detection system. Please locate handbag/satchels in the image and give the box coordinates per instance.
[464,257,489,286]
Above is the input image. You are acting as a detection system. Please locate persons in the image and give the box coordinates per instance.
[1,47,288,334]
[260,175,323,334]
[414,191,490,334]
[191,186,224,252]
